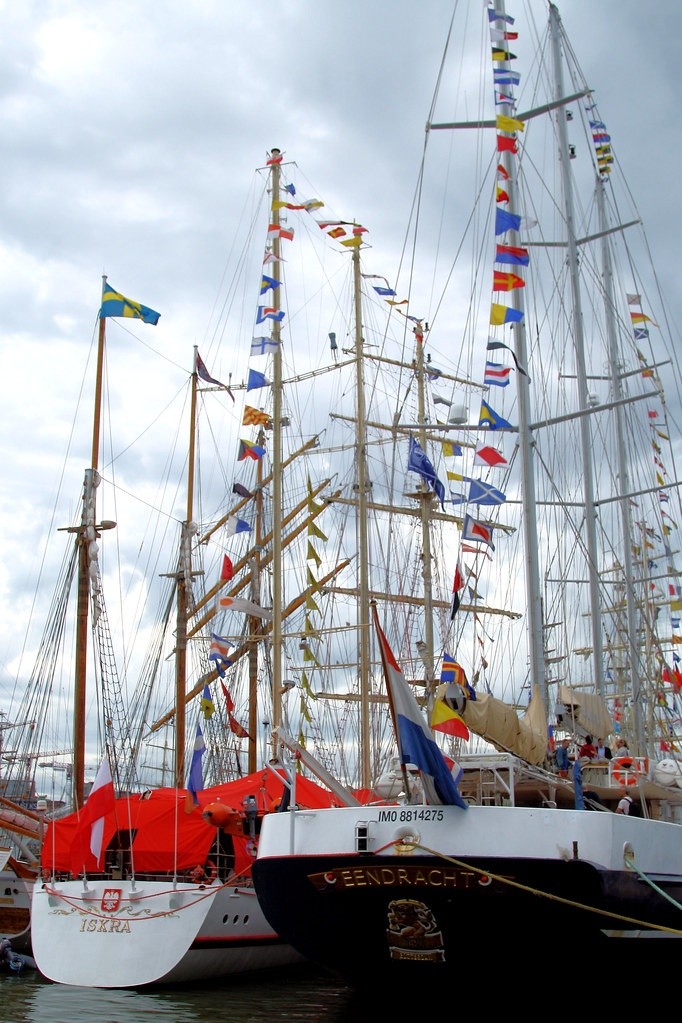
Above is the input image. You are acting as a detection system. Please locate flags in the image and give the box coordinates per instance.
[190,1,682,805]
[100,282,160,325]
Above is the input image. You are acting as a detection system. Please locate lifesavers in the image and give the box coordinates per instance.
[612,759,641,784]
[190,859,218,884]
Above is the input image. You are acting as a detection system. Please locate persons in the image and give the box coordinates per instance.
[555,735,632,816]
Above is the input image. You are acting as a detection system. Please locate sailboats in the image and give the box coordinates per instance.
[1,0,682,1005]
